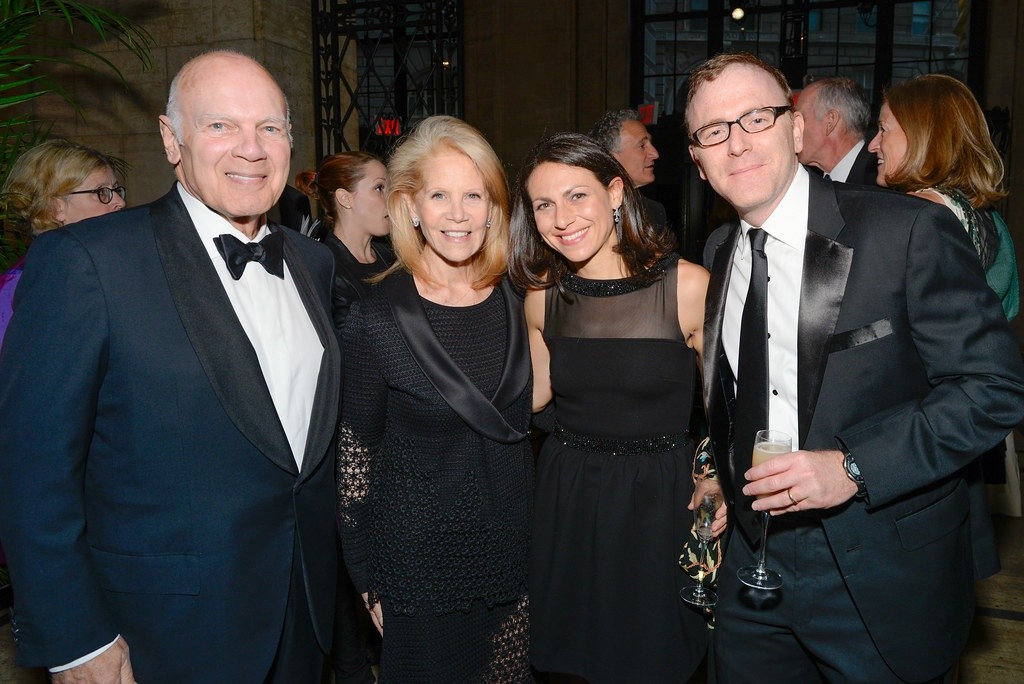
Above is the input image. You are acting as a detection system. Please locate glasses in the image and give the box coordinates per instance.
[688,104,794,147]
[63,185,128,204]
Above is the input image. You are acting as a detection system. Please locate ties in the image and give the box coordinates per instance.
[732,228,769,552]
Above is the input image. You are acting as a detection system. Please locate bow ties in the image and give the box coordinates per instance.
[216,230,286,278]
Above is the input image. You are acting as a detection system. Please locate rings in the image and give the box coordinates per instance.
[786,487,798,506]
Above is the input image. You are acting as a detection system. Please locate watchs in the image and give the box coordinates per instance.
[837,444,868,502]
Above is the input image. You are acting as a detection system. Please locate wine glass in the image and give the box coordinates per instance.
[737,430,792,589]
[679,475,719,606]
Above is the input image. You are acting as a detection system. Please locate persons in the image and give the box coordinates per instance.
[265,146,314,240]
[793,75,878,186]
[299,150,396,301]
[508,135,729,684]
[0,52,351,684]
[675,54,1023,683]
[589,106,667,237]
[867,73,1021,578]
[334,117,535,684]
[0,138,132,349]
